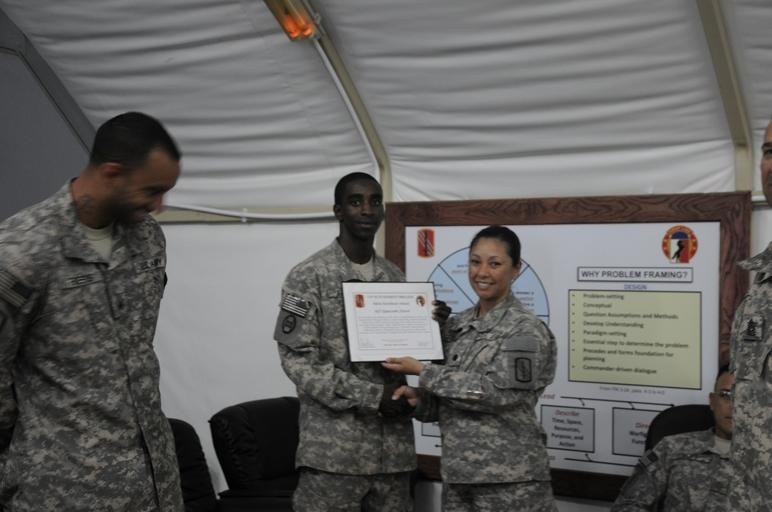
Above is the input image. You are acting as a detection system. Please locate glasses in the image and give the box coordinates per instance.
[715,390,730,400]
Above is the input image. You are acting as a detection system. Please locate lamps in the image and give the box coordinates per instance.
[261,1,325,45]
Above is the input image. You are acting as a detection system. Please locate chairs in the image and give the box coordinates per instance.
[208,394,301,512]
[166,416,220,512]
[643,405,715,452]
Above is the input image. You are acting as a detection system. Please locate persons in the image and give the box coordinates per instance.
[1,112,189,511]
[728,122,771,511]
[611,365,734,511]
[380,222,557,511]
[275,173,451,511]
